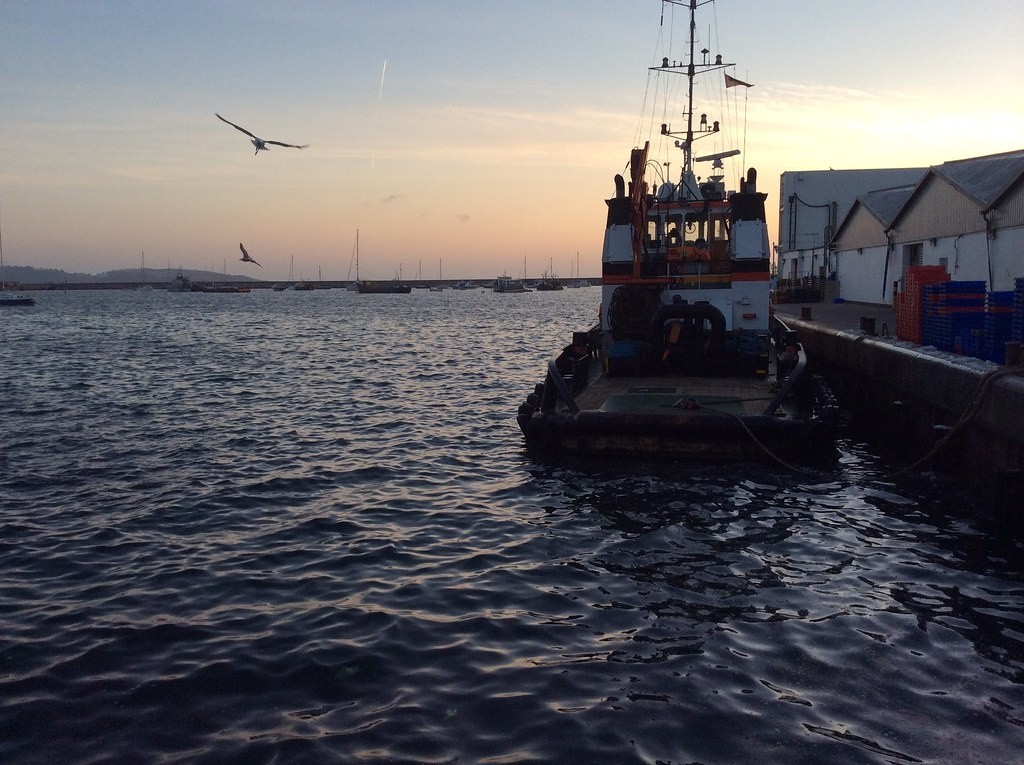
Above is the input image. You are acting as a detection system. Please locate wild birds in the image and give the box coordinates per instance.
[239,243,263,268]
[215,113,308,155]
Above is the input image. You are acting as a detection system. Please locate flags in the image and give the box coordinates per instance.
[725,73,754,88]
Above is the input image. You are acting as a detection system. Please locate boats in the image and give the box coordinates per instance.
[427,269,592,292]
[516,0,845,468]
[270,255,333,292]
[167,268,251,292]
[492,271,534,294]
[357,282,412,294]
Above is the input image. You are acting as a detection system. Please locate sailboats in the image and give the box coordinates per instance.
[0,232,36,307]
[347,230,361,292]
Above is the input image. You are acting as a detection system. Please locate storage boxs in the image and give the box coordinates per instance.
[895,264,1024,366]
